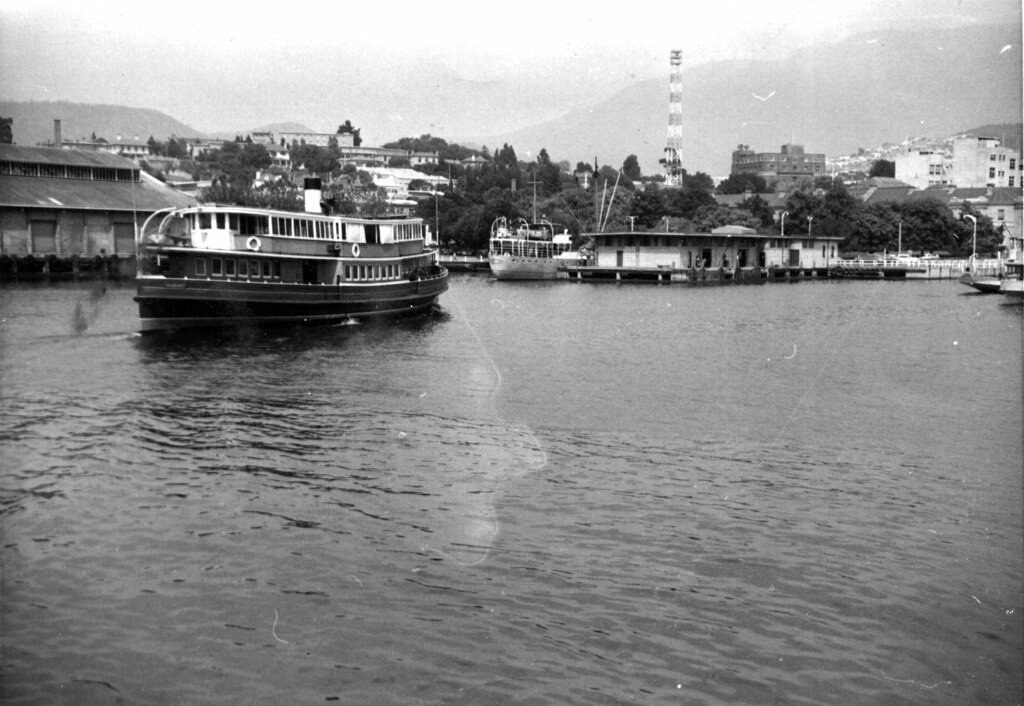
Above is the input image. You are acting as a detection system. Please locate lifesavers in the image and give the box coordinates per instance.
[352,244,360,257]
[246,236,261,252]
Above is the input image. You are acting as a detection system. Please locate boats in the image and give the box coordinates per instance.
[488,216,594,281]
[133,196,449,335]
[959,236,1024,292]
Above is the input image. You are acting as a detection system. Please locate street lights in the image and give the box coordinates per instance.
[807,216,812,235]
[662,216,668,233]
[963,215,976,259]
[627,216,638,232]
[781,211,789,236]
